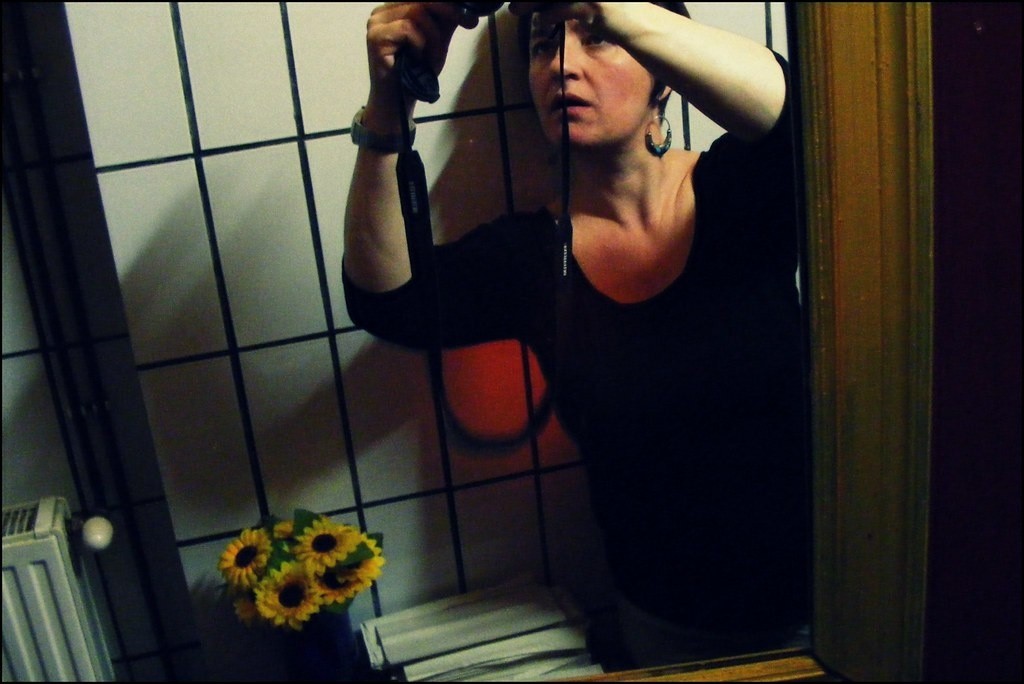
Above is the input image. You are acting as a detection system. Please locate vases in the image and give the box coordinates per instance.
[281,614,362,682]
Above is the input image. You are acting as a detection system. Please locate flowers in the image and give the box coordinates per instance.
[219,511,386,633]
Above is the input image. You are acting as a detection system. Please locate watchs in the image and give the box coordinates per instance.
[350,105,417,154]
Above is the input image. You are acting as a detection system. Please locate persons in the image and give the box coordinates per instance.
[339,1,812,672]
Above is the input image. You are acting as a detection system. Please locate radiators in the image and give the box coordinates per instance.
[2,497,118,682]
